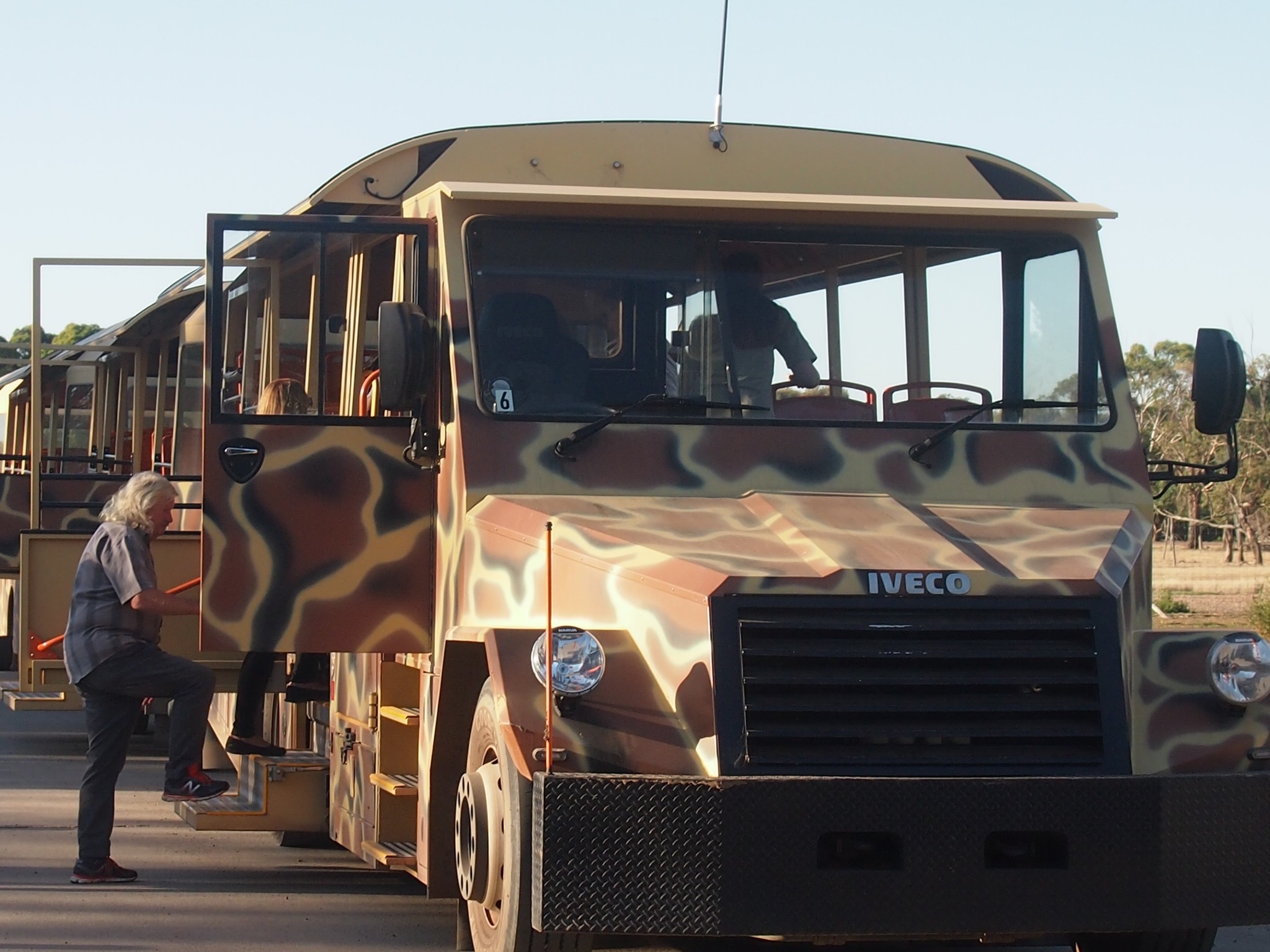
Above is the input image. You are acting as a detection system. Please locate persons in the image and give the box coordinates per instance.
[684,251,822,415]
[61,472,230,883]
[223,378,330,757]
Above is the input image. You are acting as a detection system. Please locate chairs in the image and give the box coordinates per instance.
[17,352,380,474]
[487,288,593,407]
[772,379,877,421]
[882,382,994,424]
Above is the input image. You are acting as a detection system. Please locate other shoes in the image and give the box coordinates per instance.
[285,686,330,704]
[225,737,286,756]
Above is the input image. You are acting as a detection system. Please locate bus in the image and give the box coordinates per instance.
[1,118,1269,952]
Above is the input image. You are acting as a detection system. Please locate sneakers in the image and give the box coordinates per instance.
[162,763,230,802]
[70,858,138,883]
